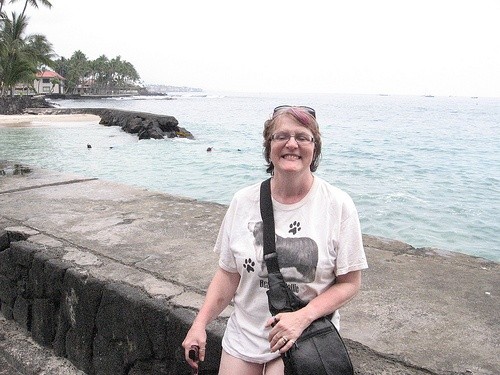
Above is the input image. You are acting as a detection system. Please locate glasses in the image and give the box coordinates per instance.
[269,132,316,144]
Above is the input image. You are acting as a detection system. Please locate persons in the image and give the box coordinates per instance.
[182,104,369,375]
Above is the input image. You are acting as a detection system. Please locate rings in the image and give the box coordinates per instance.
[281,337,287,343]
[290,340,295,344]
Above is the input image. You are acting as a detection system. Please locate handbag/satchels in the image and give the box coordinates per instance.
[267,286,354,375]
